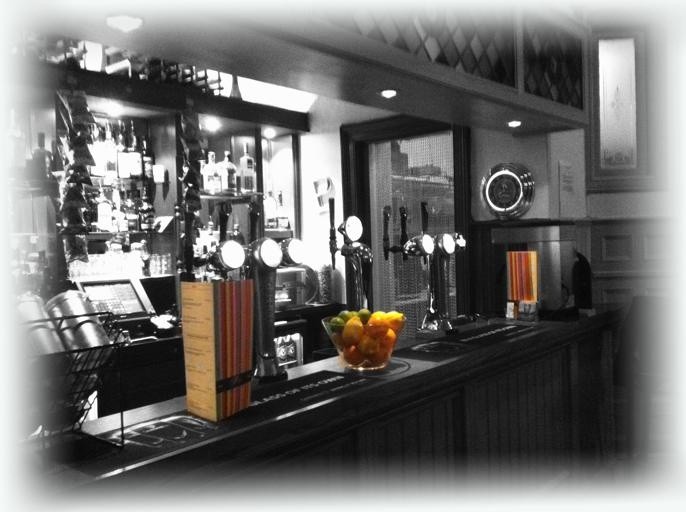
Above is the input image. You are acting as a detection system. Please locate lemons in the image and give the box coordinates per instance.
[342,310,404,344]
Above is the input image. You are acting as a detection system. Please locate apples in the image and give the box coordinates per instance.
[333,329,397,366]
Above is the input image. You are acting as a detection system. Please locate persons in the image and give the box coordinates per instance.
[572,246,597,315]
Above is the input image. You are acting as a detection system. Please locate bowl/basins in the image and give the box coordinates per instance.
[319,312,407,373]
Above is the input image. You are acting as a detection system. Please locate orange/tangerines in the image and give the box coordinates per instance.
[329,308,371,333]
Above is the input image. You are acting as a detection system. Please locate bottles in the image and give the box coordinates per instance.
[12,30,256,297]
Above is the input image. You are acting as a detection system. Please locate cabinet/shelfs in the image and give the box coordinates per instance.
[93,298,355,416]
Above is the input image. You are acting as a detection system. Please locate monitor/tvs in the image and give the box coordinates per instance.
[72,277,158,326]
